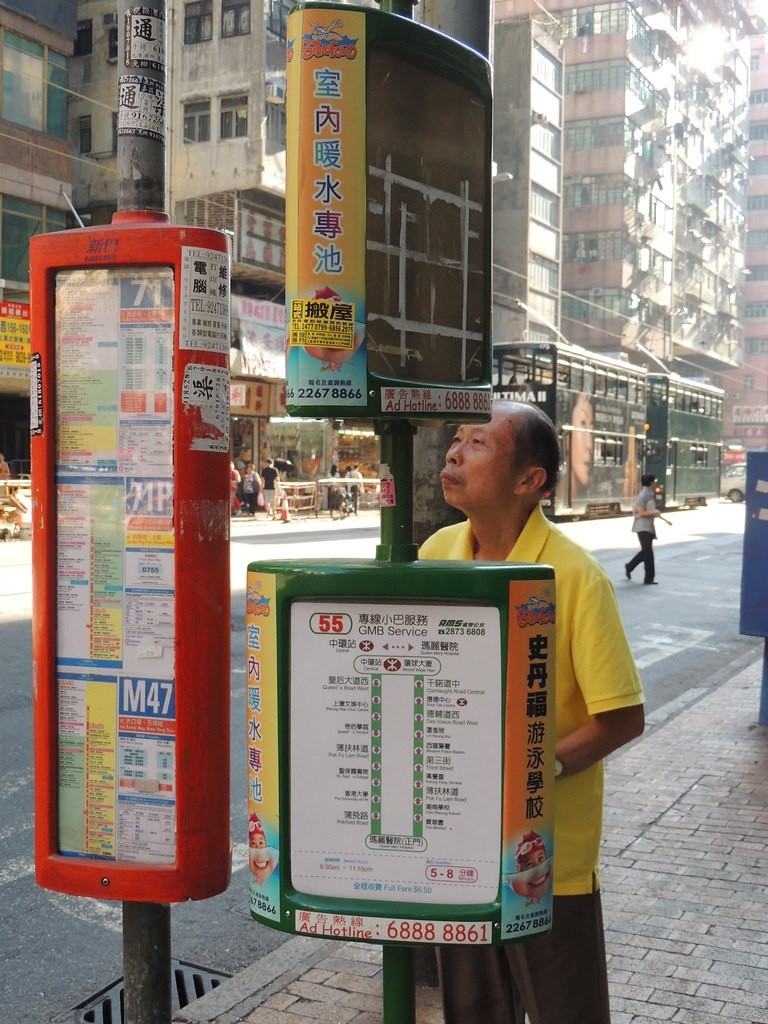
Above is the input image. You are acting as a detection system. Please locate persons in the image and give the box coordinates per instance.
[509,829,553,906]
[229,459,277,519]
[624,473,675,586]
[0,454,11,480]
[328,463,366,512]
[246,817,280,884]
[417,399,645,1024]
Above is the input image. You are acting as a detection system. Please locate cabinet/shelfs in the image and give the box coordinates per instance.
[277,481,318,518]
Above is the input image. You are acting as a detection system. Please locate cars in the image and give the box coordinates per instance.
[719,463,747,502]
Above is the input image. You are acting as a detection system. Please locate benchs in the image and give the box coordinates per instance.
[684,496,708,510]
[584,502,622,518]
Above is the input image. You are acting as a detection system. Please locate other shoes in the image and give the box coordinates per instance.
[626,563,632,579]
[644,578,659,585]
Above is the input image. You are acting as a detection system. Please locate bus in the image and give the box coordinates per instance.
[648,371,724,509]
[492,341,649,521]
